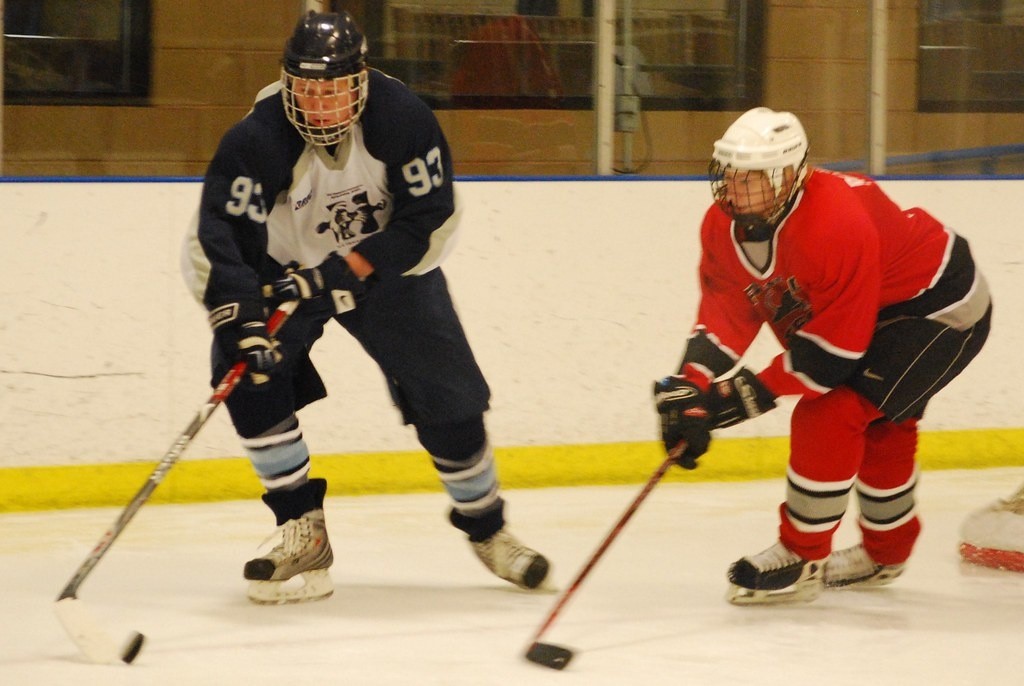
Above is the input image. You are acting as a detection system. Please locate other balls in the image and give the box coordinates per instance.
[119,633,145,664]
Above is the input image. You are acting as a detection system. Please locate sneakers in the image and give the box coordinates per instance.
[726,540,824,606]
[448,498,550,591]
[822,542,905,594]
[244,478,335,601]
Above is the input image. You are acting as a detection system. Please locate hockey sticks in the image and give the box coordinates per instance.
[522,437,691,673]
[49,297,303,667]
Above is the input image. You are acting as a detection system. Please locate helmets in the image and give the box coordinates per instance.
[278,9,368,146]
[708,106,810,230]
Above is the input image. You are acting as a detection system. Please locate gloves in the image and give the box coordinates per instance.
[275,250,358,337]
[225,310,287,396]
[654,376,712,470]
[705,365,777,432]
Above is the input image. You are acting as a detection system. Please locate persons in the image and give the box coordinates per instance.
[652,107,994,592]
[183,9,549,588]
